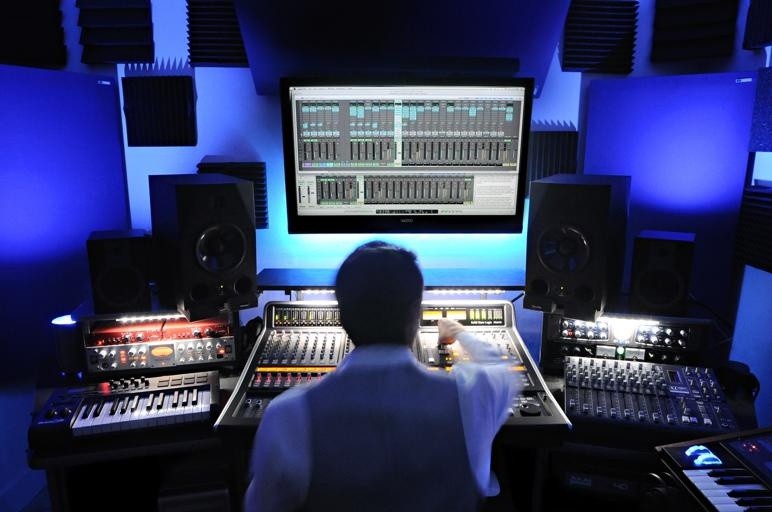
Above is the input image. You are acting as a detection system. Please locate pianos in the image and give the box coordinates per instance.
[28,370,220,451]
[655,428,770,512]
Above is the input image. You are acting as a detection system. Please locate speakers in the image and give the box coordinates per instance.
[148,173,258,322]
[85,228,151,315]
[630,229,693,317]
[523,174,631,323]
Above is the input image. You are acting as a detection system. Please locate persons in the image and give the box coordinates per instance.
[239,237,526,510]
